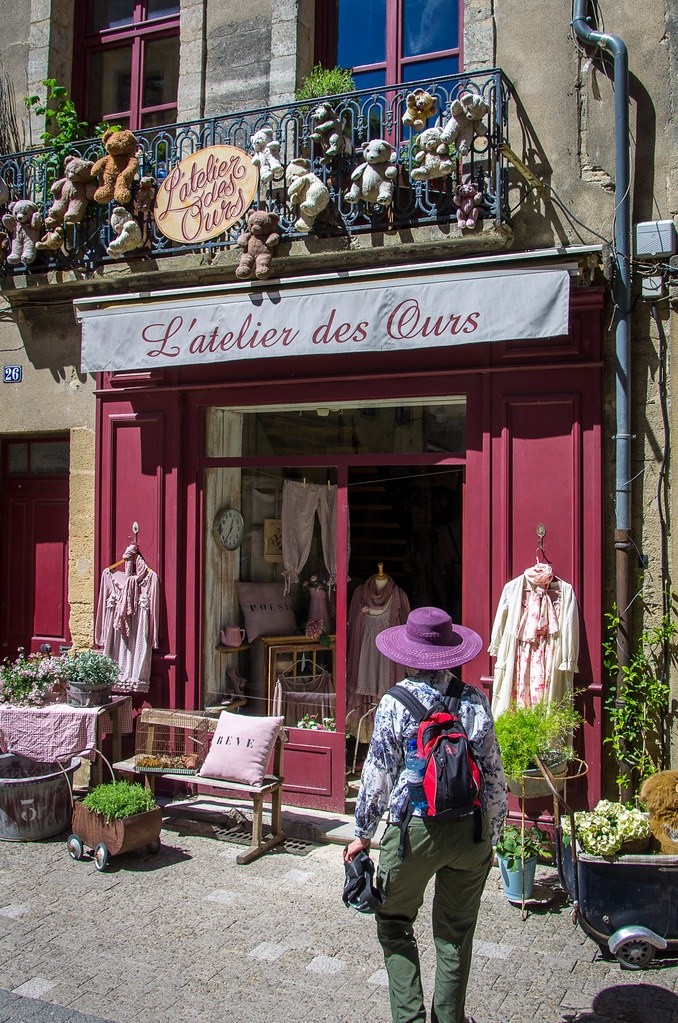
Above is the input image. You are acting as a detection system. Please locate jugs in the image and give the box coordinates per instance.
[220,622,245,647]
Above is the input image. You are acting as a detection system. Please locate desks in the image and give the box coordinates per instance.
[0,695,133,794]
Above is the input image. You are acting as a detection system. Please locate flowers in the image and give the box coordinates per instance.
[0,647,119,708]
[562,798,654,855]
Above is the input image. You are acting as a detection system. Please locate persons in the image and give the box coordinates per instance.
[341,605,509,1023]
[346,562,418,773]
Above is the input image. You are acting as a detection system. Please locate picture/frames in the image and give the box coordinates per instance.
[263,517,284,563]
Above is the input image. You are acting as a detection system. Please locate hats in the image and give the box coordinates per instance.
[375,607,483,670]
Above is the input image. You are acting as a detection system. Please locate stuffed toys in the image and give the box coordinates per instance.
[306,101,346,155]
[343,139,397,206]
[284,157,330,232]
[250,127,284,184]
[0,130,156,264]
[639,769,678,854]
[401,87,437,130]
[235,208,280,279]
[440,93,491,156]
[453,182,482,229]
[410,127,454,181]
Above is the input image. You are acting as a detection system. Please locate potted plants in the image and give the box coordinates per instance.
[492,824,554,901]
[493,687,585,798]
[66,778,161,871]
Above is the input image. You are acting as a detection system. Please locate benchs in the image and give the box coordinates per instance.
[113,708,289,866]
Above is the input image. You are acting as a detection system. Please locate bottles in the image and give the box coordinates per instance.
[405,739,427,809]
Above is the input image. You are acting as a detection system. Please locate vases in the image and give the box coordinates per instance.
[65,680,112,707]
[617,837,650,856]
[0,751,81,841]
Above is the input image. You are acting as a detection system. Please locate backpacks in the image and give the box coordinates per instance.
[383,677,488,859]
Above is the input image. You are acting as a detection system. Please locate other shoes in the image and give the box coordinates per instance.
[221,689,235,706]
[227,666,247,701]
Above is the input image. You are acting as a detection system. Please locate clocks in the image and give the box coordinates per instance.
[215,507,246,552]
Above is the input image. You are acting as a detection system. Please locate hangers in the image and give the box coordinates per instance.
[280,652,325,679]
[522,547,562,593]
[107,540,152,576]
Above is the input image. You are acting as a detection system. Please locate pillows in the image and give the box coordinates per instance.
[236,580,296,644]
[197,710,285,789]
